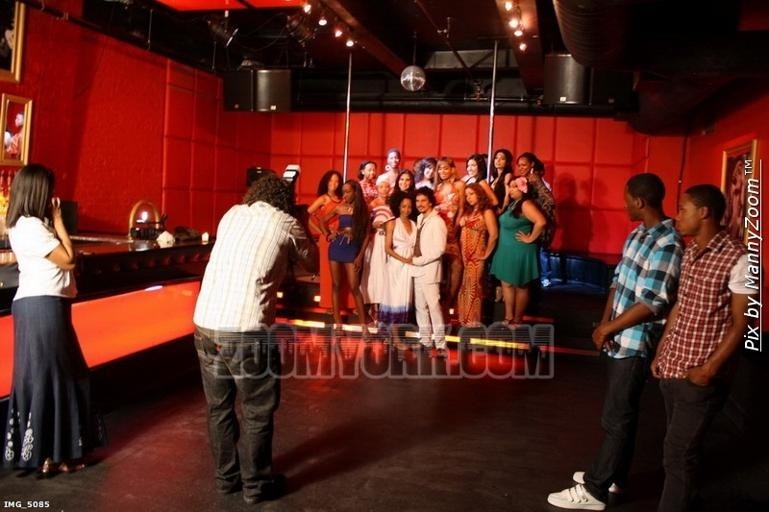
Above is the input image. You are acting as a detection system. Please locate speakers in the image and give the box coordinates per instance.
[542,53,585,105]
[223,68,291,112]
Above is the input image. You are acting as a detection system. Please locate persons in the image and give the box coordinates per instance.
[193,174,317,504]
[3,164,109,475]
[7,112,23,161]
[307,147,556,358]
[547,172,684,510]
[650,185,757,512]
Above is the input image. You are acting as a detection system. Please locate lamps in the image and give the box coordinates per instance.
[300,2,356,48]
[503,0,528,53]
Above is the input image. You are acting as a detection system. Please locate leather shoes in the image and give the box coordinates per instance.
[58,461,85,473]
[335,327,445,358]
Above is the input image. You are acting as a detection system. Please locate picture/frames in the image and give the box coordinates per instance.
[720,138,759,243]
[0,0,25,80]
[0,93,33,167]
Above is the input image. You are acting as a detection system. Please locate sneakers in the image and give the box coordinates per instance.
[547,471,614,511]
